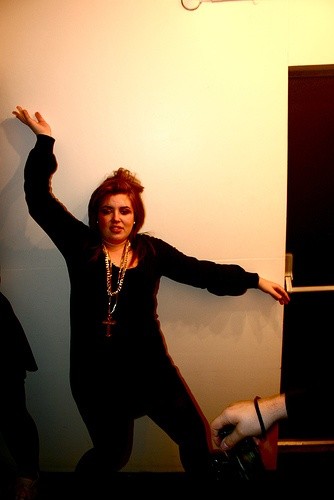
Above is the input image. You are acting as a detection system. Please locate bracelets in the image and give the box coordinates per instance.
[252,396,264,439]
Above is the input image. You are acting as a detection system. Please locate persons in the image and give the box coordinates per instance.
[14,106,290,480]
[210,384,334,449]
[0,291,40,500]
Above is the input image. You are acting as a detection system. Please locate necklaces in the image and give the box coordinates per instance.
[100,240,131,337]
